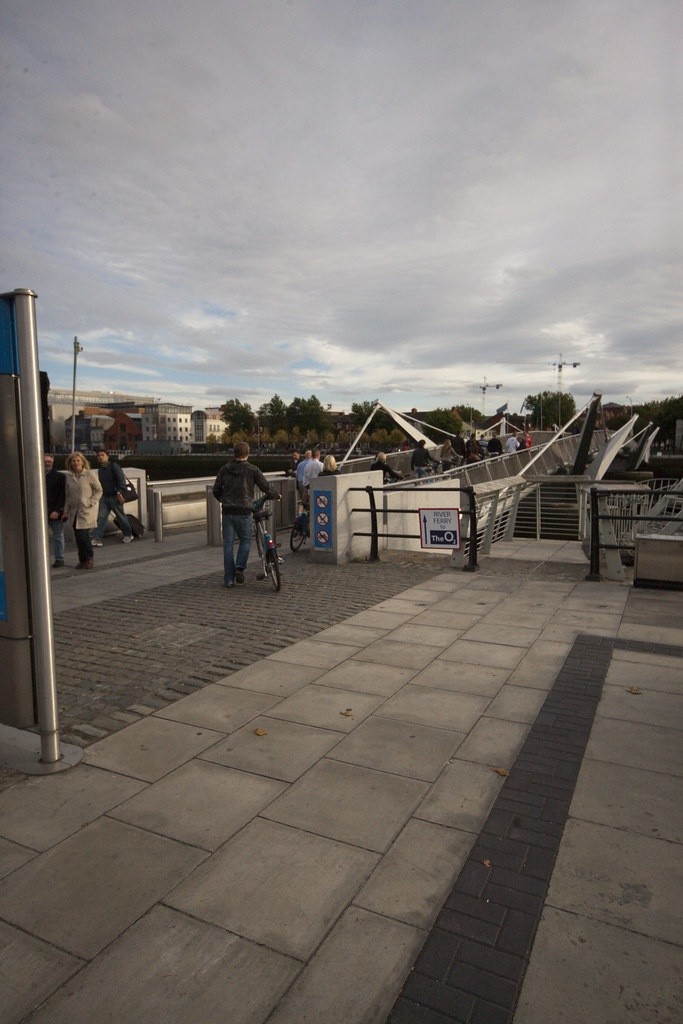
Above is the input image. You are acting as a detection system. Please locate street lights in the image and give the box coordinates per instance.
[67,334,85,455]
[625,395,634,421]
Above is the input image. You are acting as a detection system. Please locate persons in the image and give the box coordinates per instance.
[43,453,67,567]
[62,452,103,569]
[212,442,282,587]
[284,449,341,512]
[370,452,403,484]
[439,430,465,471]
[466,430,532,464]
[410,439,441,481]
[90,449,134,547]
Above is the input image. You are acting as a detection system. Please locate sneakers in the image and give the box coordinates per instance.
[122,535,134,543]
[91,539,103,547]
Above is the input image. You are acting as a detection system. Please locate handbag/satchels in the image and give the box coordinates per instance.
[469,453,481,463]
[115,484,138,504]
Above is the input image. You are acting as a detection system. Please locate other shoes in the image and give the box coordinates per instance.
[235,567,245,584]
[52,560,64,567]
[224,580,235,588]
[74,560,93,569]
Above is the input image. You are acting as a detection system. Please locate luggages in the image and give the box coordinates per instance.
[110,510,146,539]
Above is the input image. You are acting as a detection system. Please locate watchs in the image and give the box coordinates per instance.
[117,491,122,495]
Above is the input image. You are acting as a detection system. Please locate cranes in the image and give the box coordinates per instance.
[449,374,504,417]
[493,349,581,399]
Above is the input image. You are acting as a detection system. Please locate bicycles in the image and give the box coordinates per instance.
[380,455,470,484]
[288,499,311,553]
[249,492,284,593]
